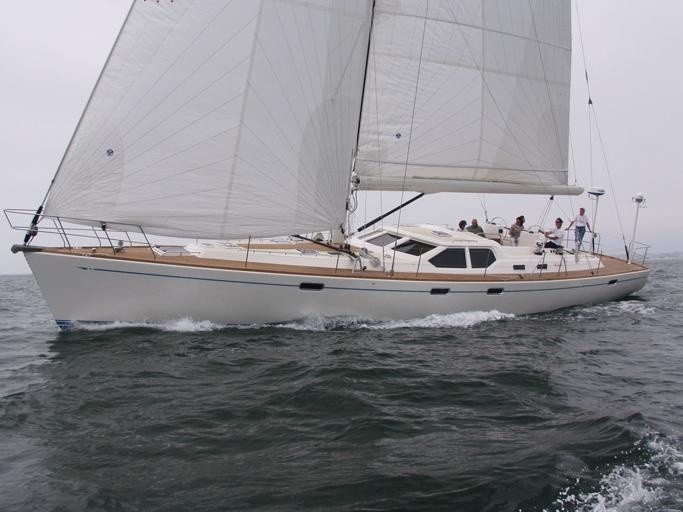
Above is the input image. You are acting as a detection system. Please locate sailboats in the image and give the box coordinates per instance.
[3,0,650,329]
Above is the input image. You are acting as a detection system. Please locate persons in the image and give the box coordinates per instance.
[457,220,467,232]
[466,218,485,237]
[565,208,592,251]
[520,215,529,232]
[534,217,565,255]
[508,217,524,244]
